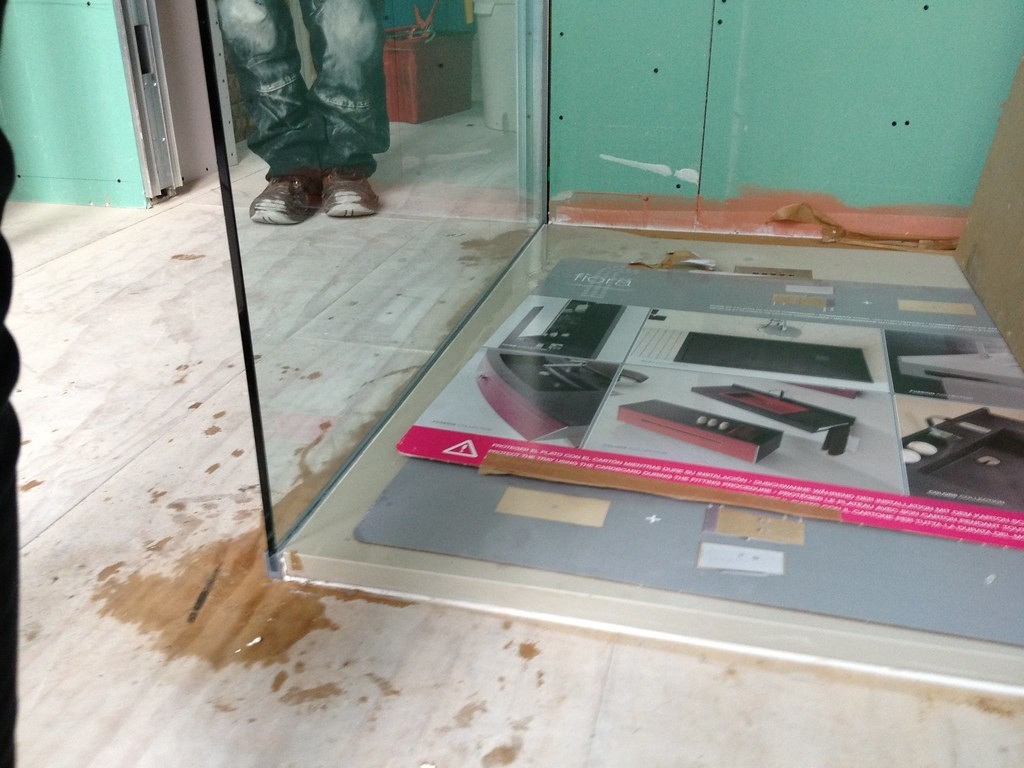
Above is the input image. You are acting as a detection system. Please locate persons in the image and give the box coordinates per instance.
[215,0,391,225]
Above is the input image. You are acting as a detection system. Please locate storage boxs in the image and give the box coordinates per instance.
[383,25,473,124]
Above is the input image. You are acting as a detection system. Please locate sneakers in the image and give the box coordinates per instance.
[319,164,380,217]
[249,174,320,224]
